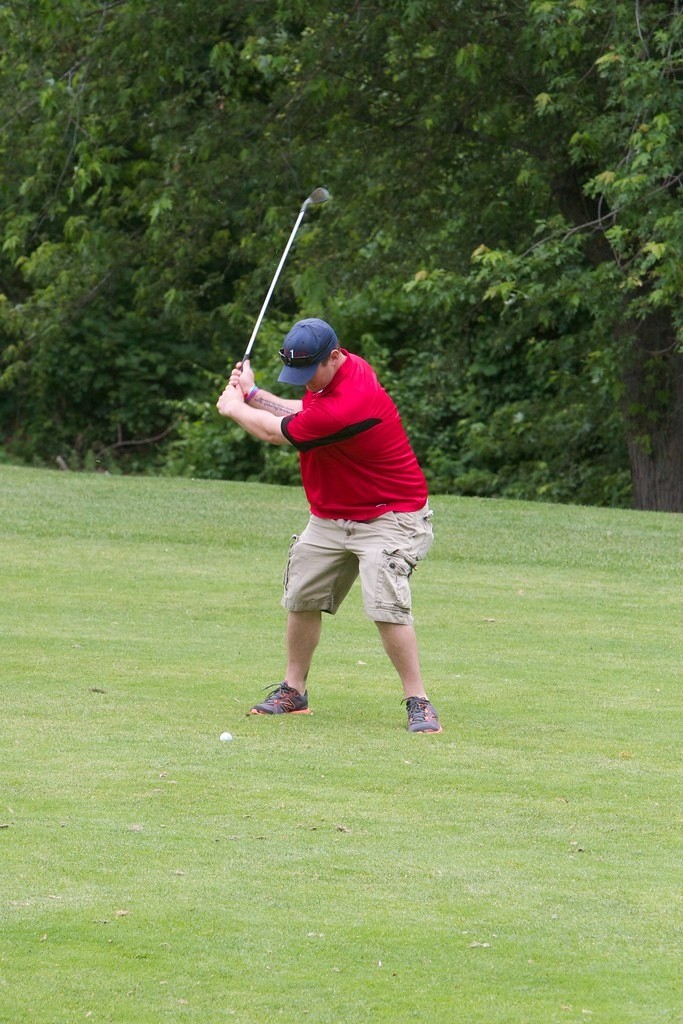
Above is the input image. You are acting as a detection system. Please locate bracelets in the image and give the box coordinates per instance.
[245,386,260,404]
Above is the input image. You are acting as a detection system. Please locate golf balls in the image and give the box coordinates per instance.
[220,732,232,742]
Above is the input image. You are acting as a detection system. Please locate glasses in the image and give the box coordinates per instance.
[278,339,331,369]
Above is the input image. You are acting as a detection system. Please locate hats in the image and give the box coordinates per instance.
[277,318,338,385]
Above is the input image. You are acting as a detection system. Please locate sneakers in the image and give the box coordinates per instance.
[249,681,310,716]
[399,696,442,734]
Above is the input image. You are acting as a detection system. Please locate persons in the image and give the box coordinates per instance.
[216,318,442,736]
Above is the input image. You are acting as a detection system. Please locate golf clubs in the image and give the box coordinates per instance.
[233,186,330,389]
